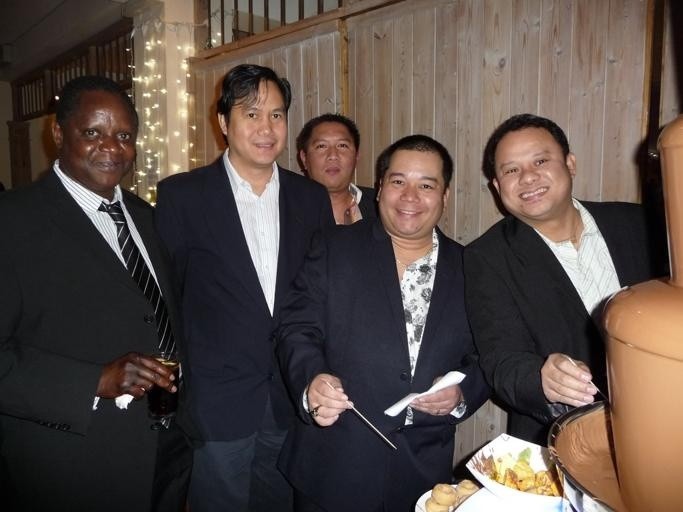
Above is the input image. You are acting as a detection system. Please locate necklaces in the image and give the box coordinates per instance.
[396,259,408,268]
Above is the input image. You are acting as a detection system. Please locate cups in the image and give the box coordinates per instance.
[146,349,182,419]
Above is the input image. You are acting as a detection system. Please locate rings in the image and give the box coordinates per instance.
[436,409,439,414]
[308,404,322,418]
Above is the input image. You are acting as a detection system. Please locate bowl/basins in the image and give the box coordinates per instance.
[545,400,618,512]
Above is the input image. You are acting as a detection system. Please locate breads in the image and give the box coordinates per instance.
[455,479,479,498]
[425,499,448,512]
[432,484,455,504]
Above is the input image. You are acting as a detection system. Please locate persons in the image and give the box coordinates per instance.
[293,112,380,227]
[268,133,494,512]
[461,112,671,448]
[149,62,336,511]
[1,72,195,510]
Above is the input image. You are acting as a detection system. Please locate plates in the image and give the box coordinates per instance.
[413,432,564,512]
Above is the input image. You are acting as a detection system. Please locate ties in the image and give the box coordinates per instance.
[99,200,182,368]
